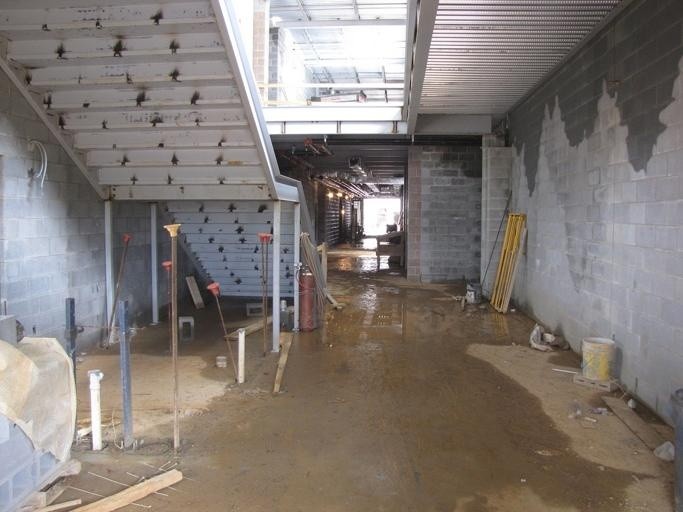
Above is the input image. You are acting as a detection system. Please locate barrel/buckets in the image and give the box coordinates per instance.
[668,388,683,511]
[580,338,616,381]
[467,283,482,304]
